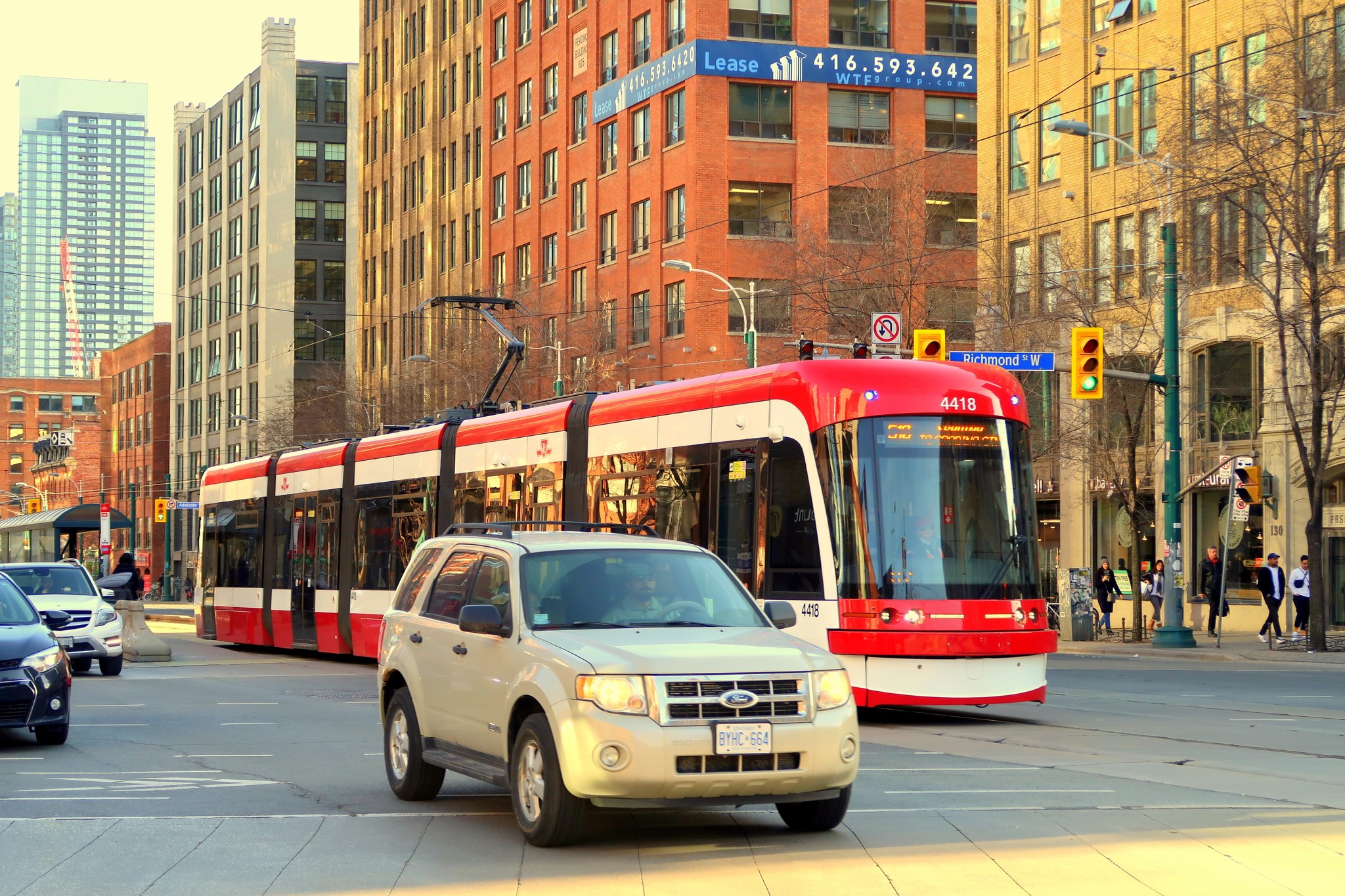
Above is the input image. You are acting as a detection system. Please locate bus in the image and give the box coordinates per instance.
[196,297,1059,708]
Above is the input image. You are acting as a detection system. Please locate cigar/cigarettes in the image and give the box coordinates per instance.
[1202,597,1202,599]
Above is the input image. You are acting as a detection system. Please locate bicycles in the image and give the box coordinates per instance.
[1042,593,1100,635]
[143,584,158,601]
[183,586,194,602]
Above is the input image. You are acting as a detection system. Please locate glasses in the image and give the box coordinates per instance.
[1302,562,1309,565]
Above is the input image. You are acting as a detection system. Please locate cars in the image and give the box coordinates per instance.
[0,572,71,746]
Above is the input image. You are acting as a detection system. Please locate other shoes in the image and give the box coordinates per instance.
[1208,630,1217,637]
[1301,636,1310,641]
[1292,632,1298,641]
[1097,624,1102,635]
[1106,628,1114,635]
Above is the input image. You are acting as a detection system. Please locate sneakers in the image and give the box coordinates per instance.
[1276,637,1287,644]
[1258,634,1268,643]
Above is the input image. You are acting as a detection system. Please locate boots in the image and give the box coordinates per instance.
[1156,620,1163,628]
[1147,618,1156,634]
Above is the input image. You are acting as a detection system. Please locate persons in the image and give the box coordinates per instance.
[137,576,145,600]
[35,577,72,594]
[1198,546,1227,637]
[1245,572,1260,590]
[598,563,682,624]
[1289,555,1311,642]
[112,553,142,601]
[184,578,192,594]
[1094,556,1124,635]
[1141,560,1165,631]
[1257,553,1286,644]
[158,573,164,588]
[525,584,542,623]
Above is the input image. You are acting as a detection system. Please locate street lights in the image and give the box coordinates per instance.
[408,356,472,409]
[48,473,90,505]
[0,490,27,515]
[16,482,54,510]
[1047,117,1196,649]
[317,386,370,437]
[662,259,779,368]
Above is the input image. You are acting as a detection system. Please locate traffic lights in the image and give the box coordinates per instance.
[853,344,866,360]
[799,340,815,361]
[1072,328,1103,401]
[1233,466,1263,506]
[914,328,946,363]
[154,499,166,522]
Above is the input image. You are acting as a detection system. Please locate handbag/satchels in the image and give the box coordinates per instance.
[1107,593,1116,603]
[1140,581,1148,595]
[1216,597,1230,617]
[1141,590,1151,601]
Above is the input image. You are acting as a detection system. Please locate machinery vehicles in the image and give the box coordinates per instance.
[57,238,84,377]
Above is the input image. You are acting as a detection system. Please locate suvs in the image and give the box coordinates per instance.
[377,521,860,849]
[0,558,123,675]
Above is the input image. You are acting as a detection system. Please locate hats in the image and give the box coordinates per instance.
[1101,556,1109,567]
[1268,553,1280,559]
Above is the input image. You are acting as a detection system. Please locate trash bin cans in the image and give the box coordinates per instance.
[170,577,181,601]
[1072,613,1094,641]
